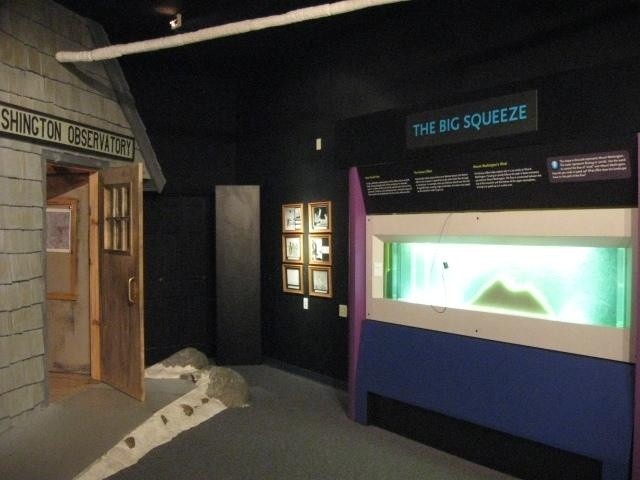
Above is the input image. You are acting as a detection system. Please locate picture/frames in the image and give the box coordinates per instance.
[282,201,333,298]
